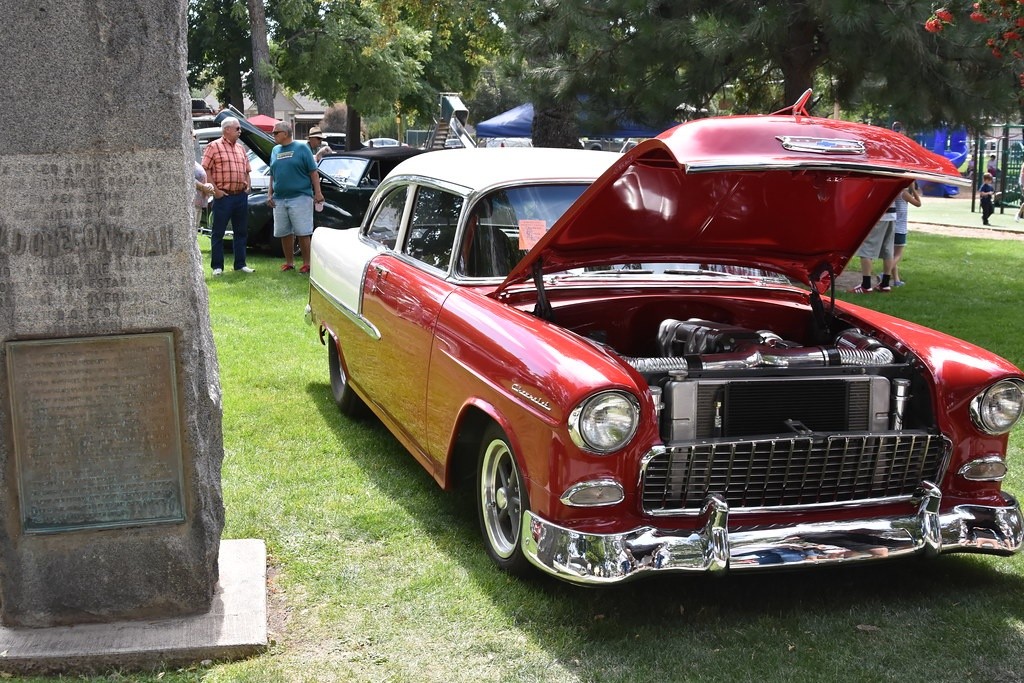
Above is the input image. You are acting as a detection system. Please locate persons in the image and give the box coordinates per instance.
[268,121,324,273]
[979,172,995,226]
[1015,151,1024,222]
[967,154,975,178]
[304,127,332,165]
[203,116,256,275]
[850,178,921,293]
[988,155,1000,177]
[194,130,215,235]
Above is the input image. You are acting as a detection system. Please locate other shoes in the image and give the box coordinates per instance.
[1015,215,1020,222]
[877,275,883,282]
[894,281,905,287]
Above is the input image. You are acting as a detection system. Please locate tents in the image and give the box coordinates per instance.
[475,77,681,153]
[247,113,292,132]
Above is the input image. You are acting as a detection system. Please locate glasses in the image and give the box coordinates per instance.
[192,135,197,139]
[232,126,242,131]
[311,137,322,141]
[272,130,285,136]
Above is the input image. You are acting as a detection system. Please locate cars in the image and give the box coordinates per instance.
[445,138,464,148]
[193,116,425,259]
[302,86,1024,595]
[584,135,638,154]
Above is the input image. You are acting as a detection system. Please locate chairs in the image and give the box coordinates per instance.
[432,190,516,278]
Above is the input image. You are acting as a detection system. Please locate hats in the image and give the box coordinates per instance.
[308,126,327,138]
[891,121,902,131]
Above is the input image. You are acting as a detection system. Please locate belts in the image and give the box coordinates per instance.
[222,188,243,195]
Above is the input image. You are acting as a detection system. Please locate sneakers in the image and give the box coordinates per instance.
[213,268,222,275]
[281,265,295,271]
[299,265,310,274]
[850,284,873,293]
[240,267,256,273]
[876,284,890,292]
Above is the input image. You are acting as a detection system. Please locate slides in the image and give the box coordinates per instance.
[449,117,478,148]
